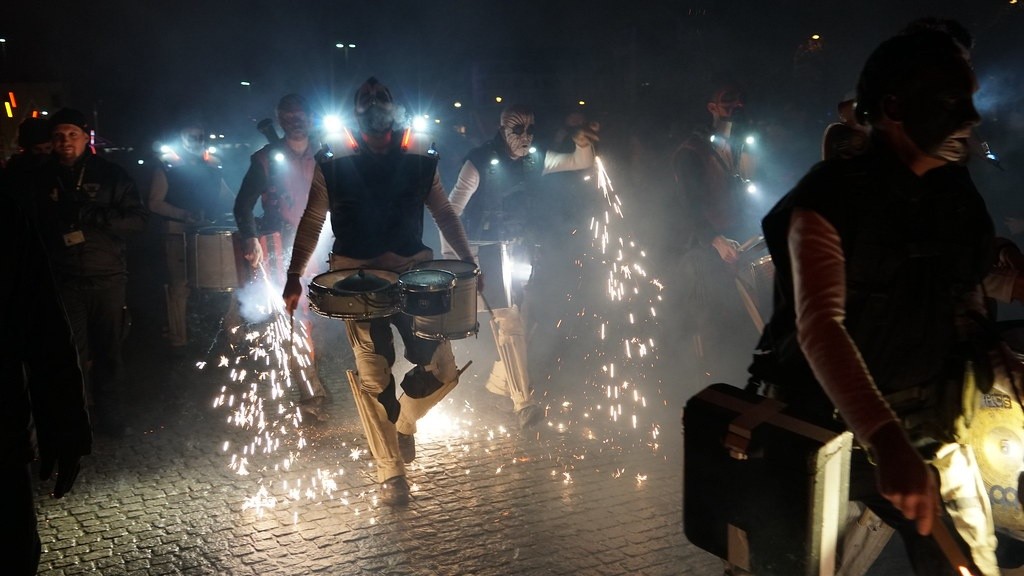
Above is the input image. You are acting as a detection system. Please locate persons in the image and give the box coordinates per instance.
[0,221,94,575]
[438,97,601,428]
[233,91,350,417]
[669,81,766,392]
[27,111,157,438]
[1,118,57,216]
[738,26,1024,576]
[148,118,243,362]
[284,75,484,509]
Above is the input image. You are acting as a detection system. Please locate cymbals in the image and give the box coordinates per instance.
[333,272,393,295]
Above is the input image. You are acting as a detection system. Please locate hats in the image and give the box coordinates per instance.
[49,108,91,137]
[19,117,54,148]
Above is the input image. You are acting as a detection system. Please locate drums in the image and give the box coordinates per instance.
[307,266,400,322]
[399,259,481,344]
[183,225,242,294]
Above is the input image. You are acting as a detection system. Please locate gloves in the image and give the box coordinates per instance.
[68,185,91,217]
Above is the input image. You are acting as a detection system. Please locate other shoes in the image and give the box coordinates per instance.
[517,400,544,429]
[379,474,410,505]
[396,432,416,463]
